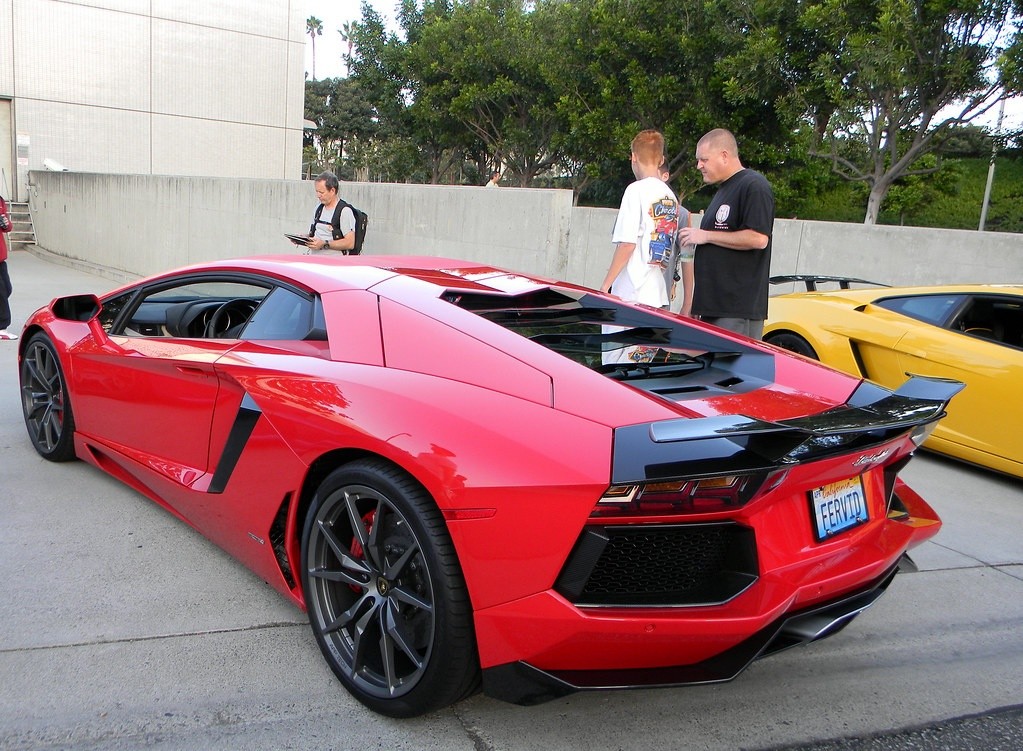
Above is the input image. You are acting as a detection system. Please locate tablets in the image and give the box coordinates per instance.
[284,233,313,246]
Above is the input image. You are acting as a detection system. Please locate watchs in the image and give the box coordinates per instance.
[323,240,329,249]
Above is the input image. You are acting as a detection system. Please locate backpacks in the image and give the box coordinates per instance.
[310,199,368,256]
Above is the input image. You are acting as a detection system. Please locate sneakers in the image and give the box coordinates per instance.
[0,329,18,339]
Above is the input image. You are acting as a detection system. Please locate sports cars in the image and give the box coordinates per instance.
[760,273,1023,481]
[17,254,971,721]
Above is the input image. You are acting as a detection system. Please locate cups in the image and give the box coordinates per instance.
[681,238,694,262]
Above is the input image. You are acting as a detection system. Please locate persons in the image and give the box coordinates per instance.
[0,196,18,340]
[677,129,775,340]
[611,158,694,315]
[600,130,680,308]
[291,172,355,255]
[486,172,500,187]
[602,324,671,366]
